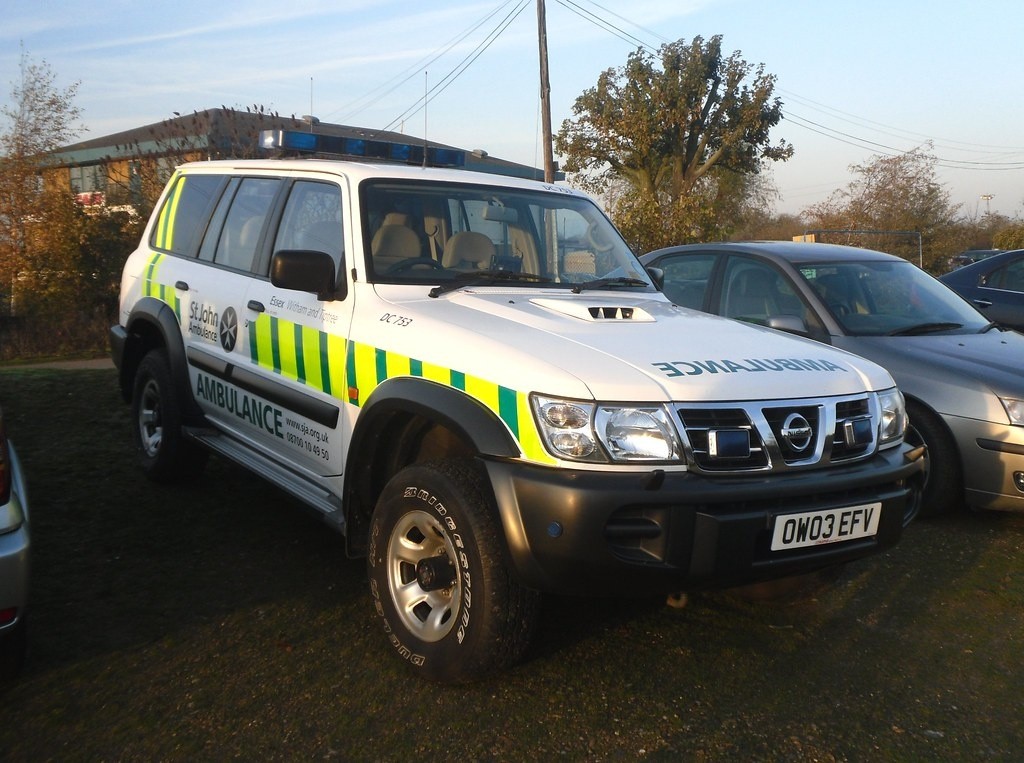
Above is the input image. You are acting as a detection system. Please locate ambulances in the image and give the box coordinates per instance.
[109,129,933,684]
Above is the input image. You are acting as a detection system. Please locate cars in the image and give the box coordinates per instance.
[598,241,1024,514]
[0,424,35,641]
[908,251,1023,335]
[933,249,1015,287]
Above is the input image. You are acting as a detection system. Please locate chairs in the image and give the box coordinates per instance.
[661,269,850,319]
[235,212,496,273]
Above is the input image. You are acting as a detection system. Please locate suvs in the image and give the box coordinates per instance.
[8,198,151,321]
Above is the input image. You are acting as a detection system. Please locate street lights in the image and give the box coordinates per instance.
[981,196,995,216]
